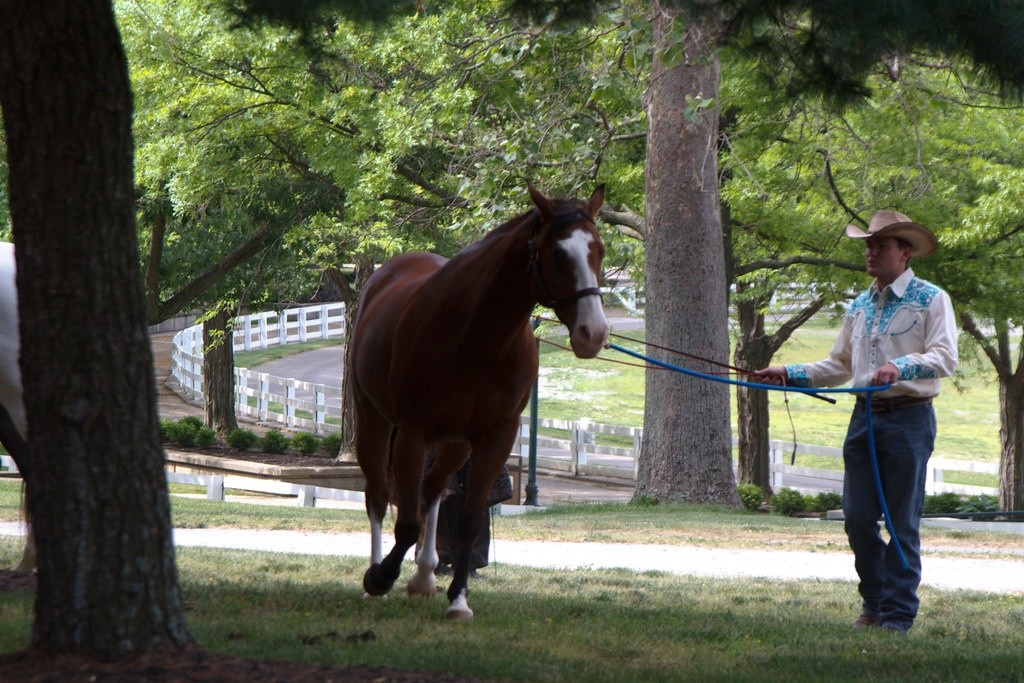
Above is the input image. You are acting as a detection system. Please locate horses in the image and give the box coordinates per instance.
[350,179,607,621]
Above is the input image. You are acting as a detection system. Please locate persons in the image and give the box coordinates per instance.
[415,466,514,578]
[754,209,959,635]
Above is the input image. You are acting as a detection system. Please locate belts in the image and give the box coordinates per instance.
[856,395,933,412]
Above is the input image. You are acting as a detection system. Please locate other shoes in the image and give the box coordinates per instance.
[882,626,907,637]
[853,614,881,628]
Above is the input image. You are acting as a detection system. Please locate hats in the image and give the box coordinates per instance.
[846,210,938,258]
